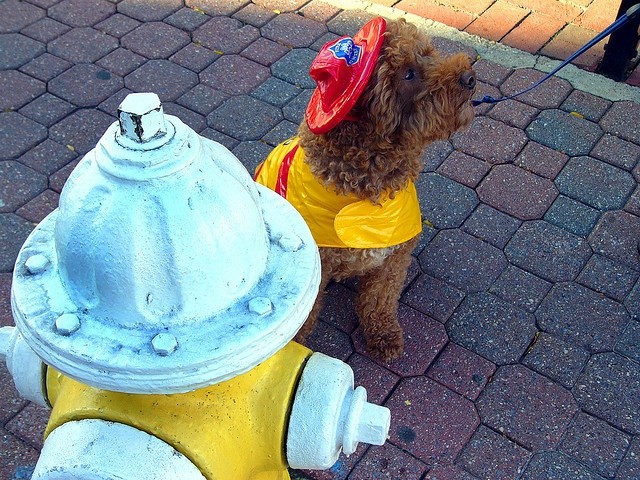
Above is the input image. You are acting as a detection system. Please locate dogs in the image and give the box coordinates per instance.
[255,18,477,359]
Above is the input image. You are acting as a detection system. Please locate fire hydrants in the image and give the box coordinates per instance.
[1,92,392,476]
